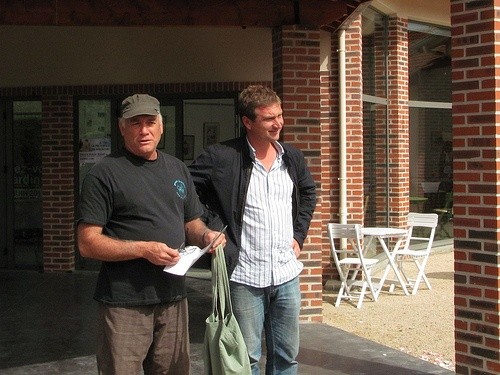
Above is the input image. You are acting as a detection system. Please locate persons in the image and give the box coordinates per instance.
[186,83,317,375]
[76,94,226,375]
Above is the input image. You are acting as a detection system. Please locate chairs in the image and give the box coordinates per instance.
[326,223,380,311]
[432,195,456,242]
[389,212,438,295]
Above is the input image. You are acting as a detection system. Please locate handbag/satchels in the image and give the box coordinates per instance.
[202,245,254,374]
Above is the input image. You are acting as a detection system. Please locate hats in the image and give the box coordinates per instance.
[120,94,161,118]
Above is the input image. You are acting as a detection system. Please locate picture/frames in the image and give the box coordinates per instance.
[183,135,195,160]
[158,116,166,150]
[203,122,220,148]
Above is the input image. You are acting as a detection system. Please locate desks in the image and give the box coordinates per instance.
[409,196,428,244]
[341,228,410,301]
